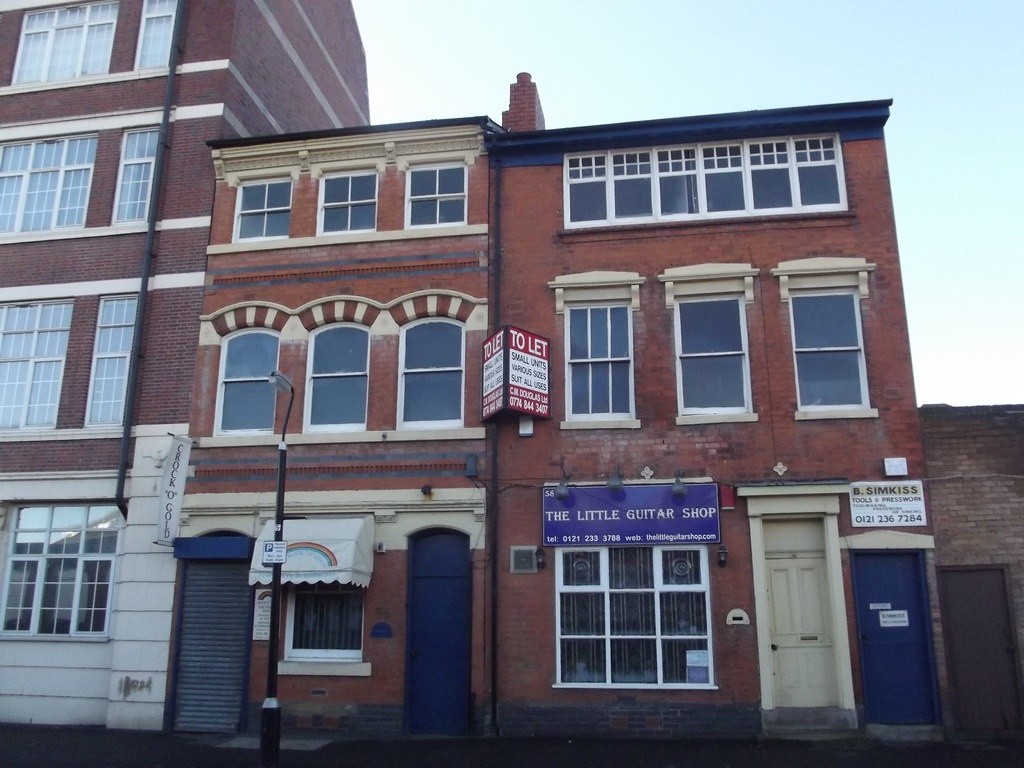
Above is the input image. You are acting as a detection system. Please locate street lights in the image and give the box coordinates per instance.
[260,370,294,768]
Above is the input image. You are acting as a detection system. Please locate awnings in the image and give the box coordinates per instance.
[246,514,376,590]
